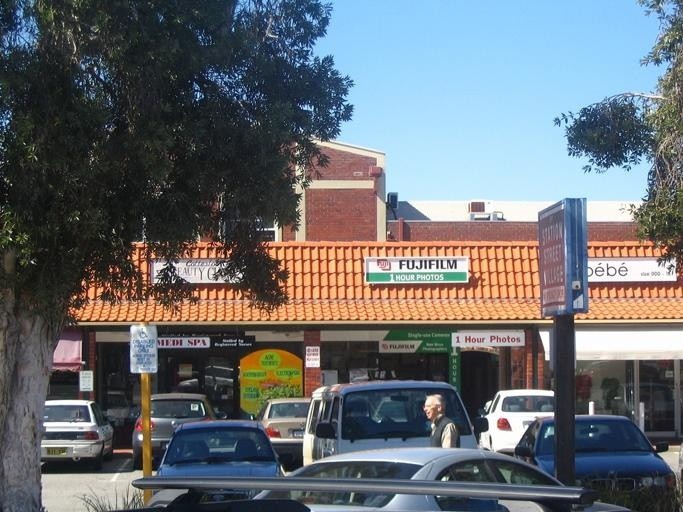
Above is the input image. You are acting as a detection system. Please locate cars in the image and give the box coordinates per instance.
[608,382,675,430]
[205,364,235,400]
[151,421,293,503]
[132,393,227,468]
[479,389,555,454]
[39,399,113,469]
[250,398,313,459]
[102,390,130,431]
[132,447,633,512]
[512,416,676,500]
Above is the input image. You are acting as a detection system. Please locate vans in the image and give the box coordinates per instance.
[303,380,489,466]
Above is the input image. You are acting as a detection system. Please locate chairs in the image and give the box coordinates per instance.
[540,404,554,411]
[234,437,259,459]
[182,441,209,461]
[504,404,521,411]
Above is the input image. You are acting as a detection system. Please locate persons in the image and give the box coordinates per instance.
[423,393,460,503]
[611,385,628,416]
